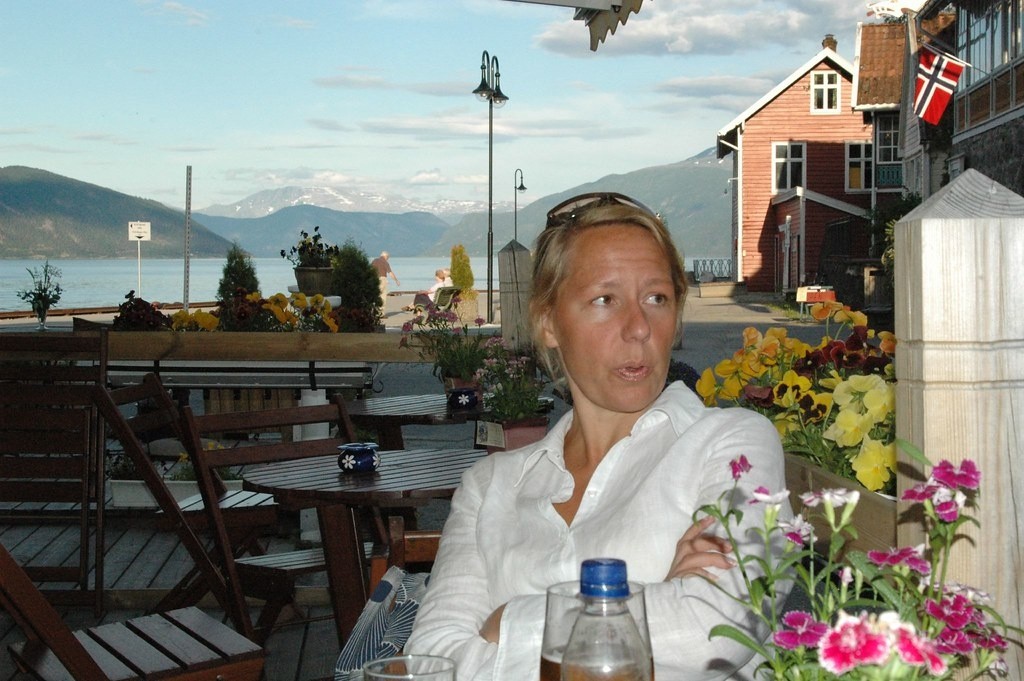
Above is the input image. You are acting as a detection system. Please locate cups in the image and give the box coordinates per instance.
[363,654,457,681]
[539,579,656,681]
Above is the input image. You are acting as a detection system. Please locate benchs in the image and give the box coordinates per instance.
[412,287,462,318]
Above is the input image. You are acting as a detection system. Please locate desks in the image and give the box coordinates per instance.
[346,393,554,573]
[242,449,488,652]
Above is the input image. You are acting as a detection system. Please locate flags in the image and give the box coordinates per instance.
[913,45,962,125]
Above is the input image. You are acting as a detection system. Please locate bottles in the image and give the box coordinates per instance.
[560,557,653,681]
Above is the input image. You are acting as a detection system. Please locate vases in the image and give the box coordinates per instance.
[480,414,551,456]
[443,377,484,401]
[784,453,897,590]
[294,266,334,297]
[301,388,330,441]
[35,307,48,331]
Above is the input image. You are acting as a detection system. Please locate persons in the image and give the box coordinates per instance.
[370,251,452,318]
[406,197,799,681]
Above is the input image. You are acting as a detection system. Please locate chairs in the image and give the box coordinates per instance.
[91,372,308,656]
[0,327,108,619]
[178,393,389,681]
[0,544,265,681]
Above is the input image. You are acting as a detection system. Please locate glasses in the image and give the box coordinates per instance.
[545,192,659,225]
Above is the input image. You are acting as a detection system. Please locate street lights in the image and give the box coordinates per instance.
[513,168,527,242]
[472,50,510,323]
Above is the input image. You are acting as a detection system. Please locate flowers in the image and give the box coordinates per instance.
[399,297,492,384]
[110,289,173,332]
[332,305,384,333]
[691,439,1024,681]
[15,258,67,311]
[474,337,548,424]
[280,225,340,267]
[169,440,242,480]
[210,287,270,332]
[262,292,339,391]
[694,301,898,497]
[171,309,221,331]
